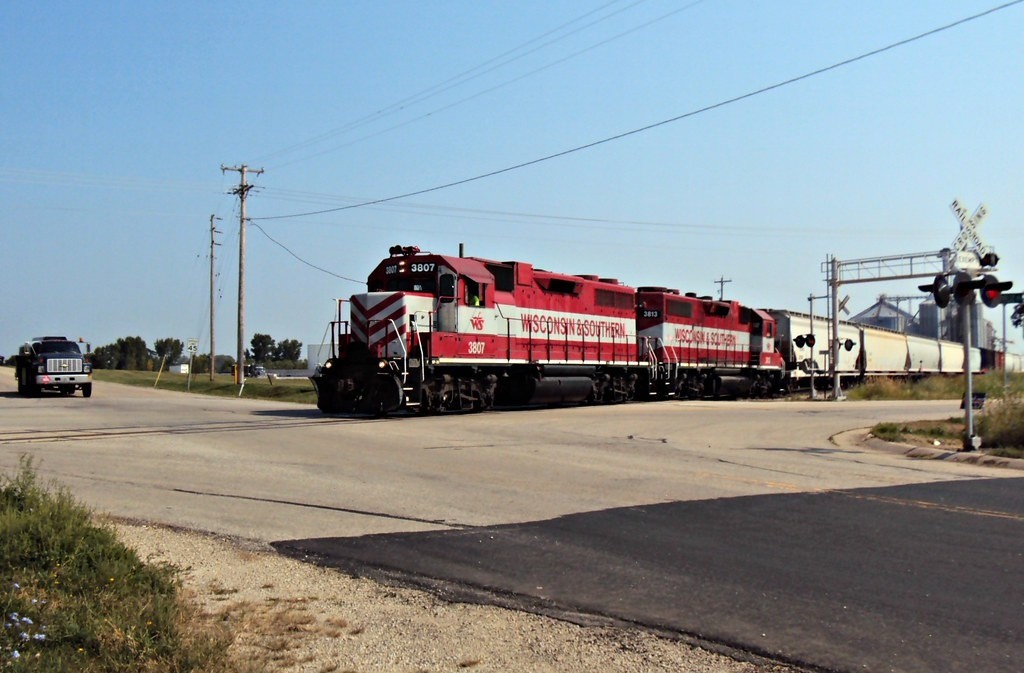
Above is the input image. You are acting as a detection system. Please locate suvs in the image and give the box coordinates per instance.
[14,335,93,398]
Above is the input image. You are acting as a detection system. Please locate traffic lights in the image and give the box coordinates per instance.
[792,335,816,350]
[837,339,857,350]
[932,272,1013,308]
[918,277,949,307]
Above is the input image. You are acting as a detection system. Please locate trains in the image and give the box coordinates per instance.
[304,239,1020,417]
